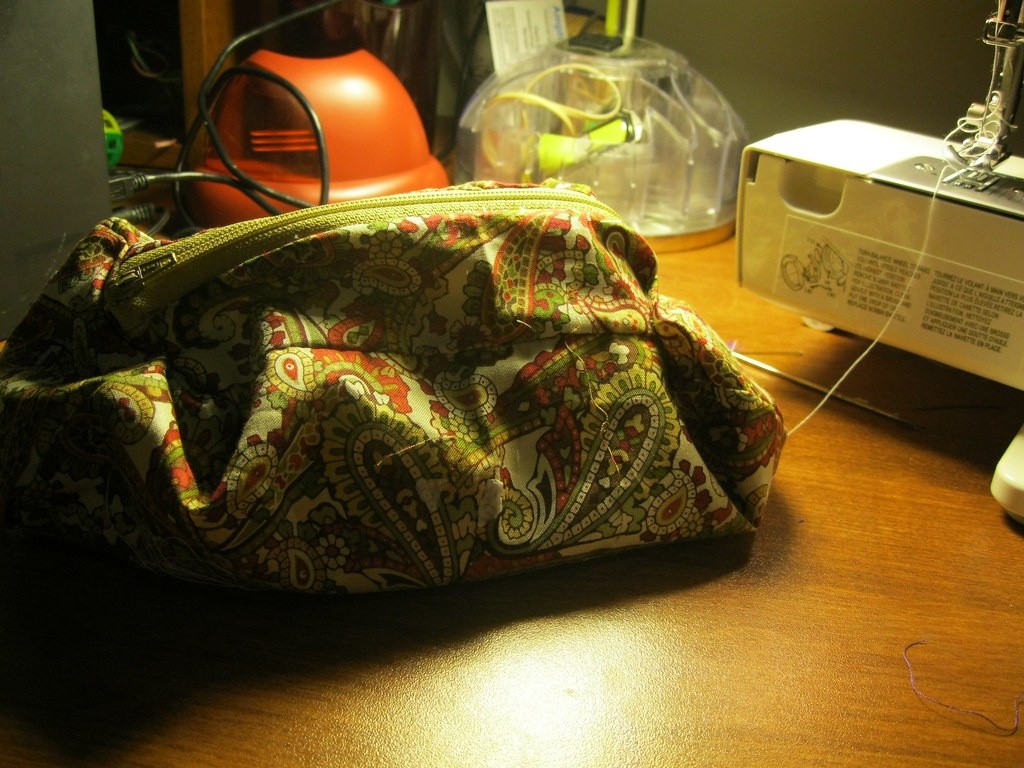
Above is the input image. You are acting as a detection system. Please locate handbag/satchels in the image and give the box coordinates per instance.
[0,178,789,596]
